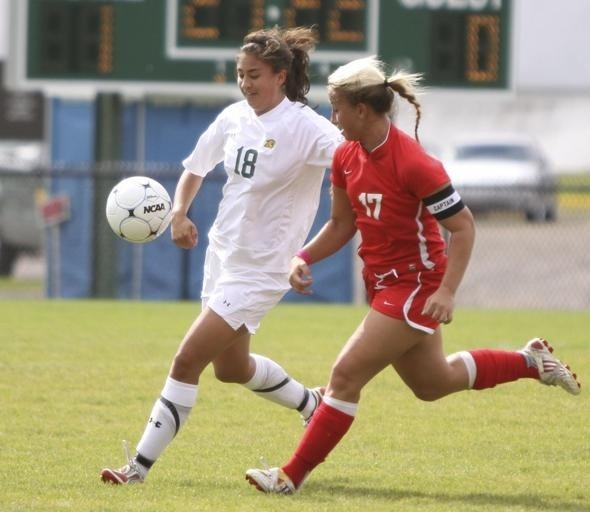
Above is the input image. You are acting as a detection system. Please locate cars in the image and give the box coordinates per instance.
[442,136,558,224]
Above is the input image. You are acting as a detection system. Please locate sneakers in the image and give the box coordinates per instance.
[244,463,296,496]
[300,385,328,429]
[522,335,582,396]
[98,457,146,488]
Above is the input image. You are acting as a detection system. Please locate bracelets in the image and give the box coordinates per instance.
[291,250,315,267]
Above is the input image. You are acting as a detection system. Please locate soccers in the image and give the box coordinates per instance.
[106,176,173,243]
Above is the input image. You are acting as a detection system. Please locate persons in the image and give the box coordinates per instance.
[101,27,347,487]
[246,56,581,493]
